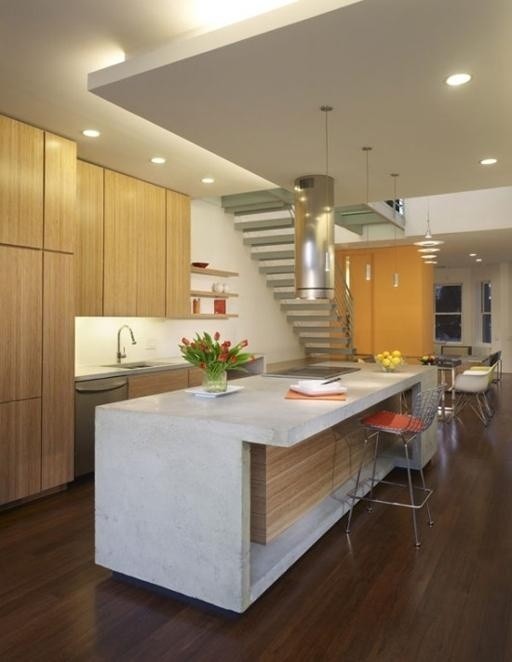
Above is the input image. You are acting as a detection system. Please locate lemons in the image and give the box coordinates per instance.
[376,350,404,370]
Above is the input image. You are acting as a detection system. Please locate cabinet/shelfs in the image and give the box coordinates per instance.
[77,158,239,320]
[0,115,77,507]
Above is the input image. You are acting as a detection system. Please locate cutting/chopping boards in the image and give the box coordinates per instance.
[285,387,346,401]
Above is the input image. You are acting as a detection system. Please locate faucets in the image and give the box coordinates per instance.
[116,322,137,363]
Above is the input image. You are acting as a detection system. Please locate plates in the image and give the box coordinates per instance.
[183,383,245,396]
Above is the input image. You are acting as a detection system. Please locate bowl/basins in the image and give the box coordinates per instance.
[373,355,407,371]
[192,262,209,269]
[290,378,346,397]
[418,359,439,365]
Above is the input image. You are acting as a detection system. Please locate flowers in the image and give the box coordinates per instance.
[179,331,256,381]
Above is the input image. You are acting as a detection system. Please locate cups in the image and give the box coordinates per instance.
[213,299,226,314]
[193,298,201,314]
[214,282,232,295]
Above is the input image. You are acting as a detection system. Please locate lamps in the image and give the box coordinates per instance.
[390,173,401,288]
[362,146,374,281]
[414,191,445,265]
[320,106,333,271]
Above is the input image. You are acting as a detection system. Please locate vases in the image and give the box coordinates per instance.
[201,372,227,391]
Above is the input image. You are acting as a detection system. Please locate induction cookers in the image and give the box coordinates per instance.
[263,365,361,380]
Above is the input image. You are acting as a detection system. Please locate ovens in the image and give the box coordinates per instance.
[69,374,128,488]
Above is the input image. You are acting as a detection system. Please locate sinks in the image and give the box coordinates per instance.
[98,359,175,371]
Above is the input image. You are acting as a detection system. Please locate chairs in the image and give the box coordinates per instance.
[449,351,502,427]
[345,384,447,545]
